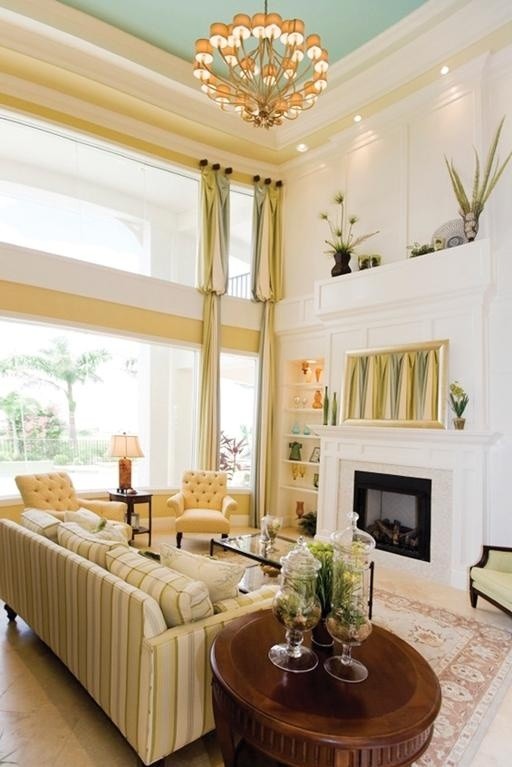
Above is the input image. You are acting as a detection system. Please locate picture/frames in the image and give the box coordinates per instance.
[341,339,449,430]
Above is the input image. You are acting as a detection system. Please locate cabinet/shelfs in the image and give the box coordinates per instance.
[276,357,327,545]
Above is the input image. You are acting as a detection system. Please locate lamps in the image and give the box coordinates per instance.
[103,433,144,493]
[193,0,329,131]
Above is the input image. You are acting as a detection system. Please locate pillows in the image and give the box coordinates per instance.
[159,540,247,601]
[64,511,97,533]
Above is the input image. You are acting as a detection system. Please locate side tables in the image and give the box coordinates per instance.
[106,488,153,547]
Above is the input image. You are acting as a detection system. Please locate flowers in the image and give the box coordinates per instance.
[448,381,470,418]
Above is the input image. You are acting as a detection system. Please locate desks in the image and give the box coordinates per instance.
[209,608,443,767]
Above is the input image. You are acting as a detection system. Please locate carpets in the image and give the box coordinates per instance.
[361,578,512,767]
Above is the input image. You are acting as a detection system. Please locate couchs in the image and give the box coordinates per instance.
[0,509,280,766]
[15,471,133,547]
[470,544,512,619]
[167,469,237,552]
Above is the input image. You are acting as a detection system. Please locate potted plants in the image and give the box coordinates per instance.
[443,116,512,242]
[285,541,364,648]
[320,192,381,277]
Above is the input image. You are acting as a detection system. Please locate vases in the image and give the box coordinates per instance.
[453,418,466,430]
